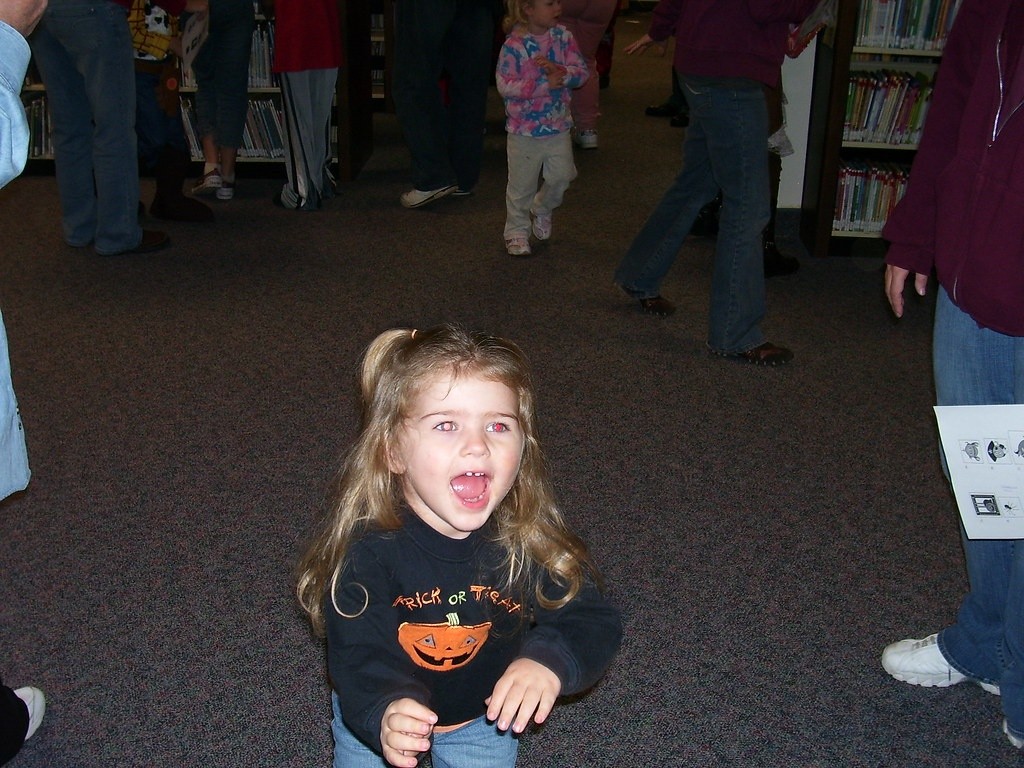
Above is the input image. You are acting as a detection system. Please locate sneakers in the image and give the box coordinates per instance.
[453,189,470,195]
[575,128,599,148]
[191,168,223,195]
[881,634,1000,696]
[530,208,552,240]
[215,177,236,200]
[1002,719,1024,753]
[400,184,458,208]
[505,238,531,256]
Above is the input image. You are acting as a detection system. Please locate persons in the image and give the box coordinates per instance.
[189,0,256,200]
[27,0,210,255]
[495,0,589,256]
[296,322,624,768]
[390,0,491,208]
[0,0,47,768]
[881,1,1024,750]
[271,0,341,210]
[557,1,628,149]
[614,1,819,367]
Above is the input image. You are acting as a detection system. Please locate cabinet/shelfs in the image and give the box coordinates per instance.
[802,1,963,260]
[6,6,341,199]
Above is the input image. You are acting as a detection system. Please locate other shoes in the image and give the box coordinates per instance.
[622,285,676,317]
[13,686,46,740]
[130,229,171,253]
[708,342,794,366]
[671,110,690,127]
[598,74,609,89]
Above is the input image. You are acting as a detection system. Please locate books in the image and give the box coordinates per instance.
[248,23,280,87]
[855,1,962,52]
[180,60,205,160]
[24,95,55,157]
[832,157,910,234]
[372,14,385,85]
[843,54,944,146]
[242,94,284,159]
